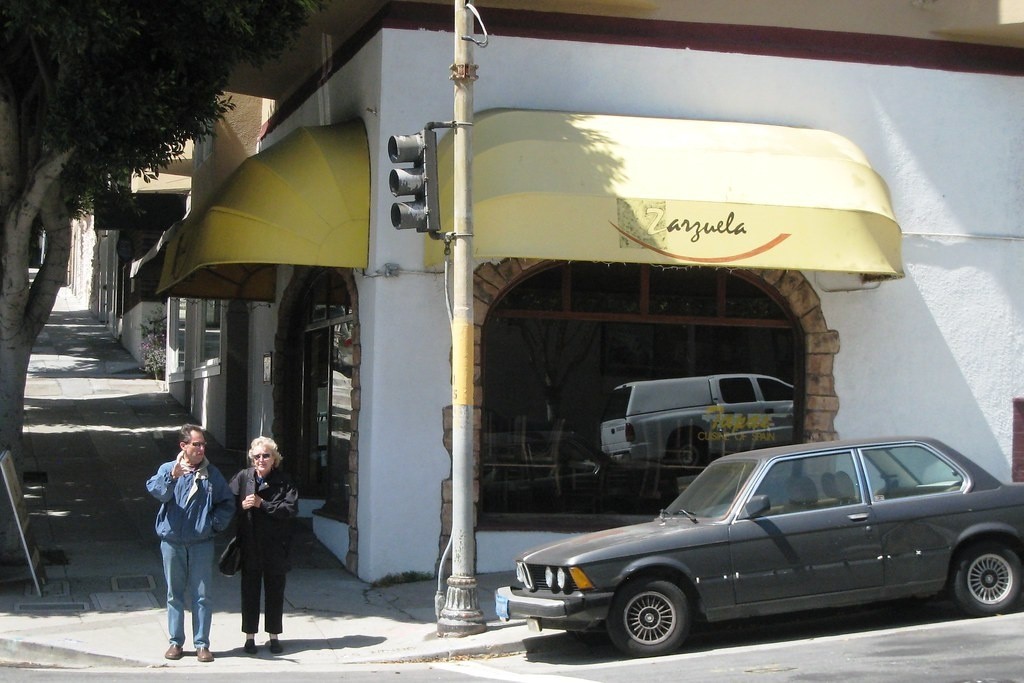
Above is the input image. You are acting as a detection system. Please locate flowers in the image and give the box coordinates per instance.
[134,313,165,370]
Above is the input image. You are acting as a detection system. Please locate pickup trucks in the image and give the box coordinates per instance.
[599,371,797,475]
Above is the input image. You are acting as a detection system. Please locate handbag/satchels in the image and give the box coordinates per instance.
[218,536,241,575]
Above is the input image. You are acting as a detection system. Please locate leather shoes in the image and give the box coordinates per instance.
[197,648,214,661]
[165,645,183,659]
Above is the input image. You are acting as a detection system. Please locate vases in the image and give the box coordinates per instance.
[155,369,165,380]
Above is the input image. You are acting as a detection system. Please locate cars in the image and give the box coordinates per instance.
[479,422,679,518]
[490,435,1024,660]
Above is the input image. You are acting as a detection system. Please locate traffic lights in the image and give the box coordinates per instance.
[386,129,442,232]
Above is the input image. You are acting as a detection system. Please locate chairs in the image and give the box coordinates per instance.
[783,471,861,514]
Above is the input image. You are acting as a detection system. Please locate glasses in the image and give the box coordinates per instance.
[187,442,207,447]
[253,453,270,460]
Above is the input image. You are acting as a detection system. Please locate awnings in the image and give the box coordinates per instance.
[127,117,372,305]
[423,109,905,294]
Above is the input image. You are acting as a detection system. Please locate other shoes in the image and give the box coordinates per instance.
[245,639,257,653]
[270,639,282,653]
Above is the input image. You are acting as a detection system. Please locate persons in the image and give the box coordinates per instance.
[146,424,236,661]
[229,436,299,654]
